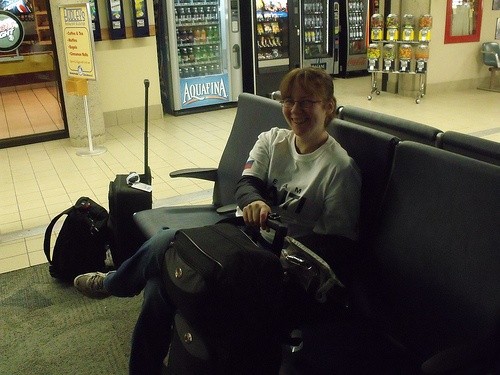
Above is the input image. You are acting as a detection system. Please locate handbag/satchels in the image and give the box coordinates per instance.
[260,225,344,308]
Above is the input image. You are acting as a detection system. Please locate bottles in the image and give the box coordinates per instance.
[255,0,288,60]
[303,0,324,58]
[174,0,220,78]
[348,0,364,55]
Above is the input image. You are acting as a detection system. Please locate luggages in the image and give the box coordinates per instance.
[107,79,153,268]
[165,212,289,375]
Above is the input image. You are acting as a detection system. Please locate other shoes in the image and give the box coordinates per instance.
[74,271,117,300]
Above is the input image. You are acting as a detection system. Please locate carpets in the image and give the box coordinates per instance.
[0,260,175,375]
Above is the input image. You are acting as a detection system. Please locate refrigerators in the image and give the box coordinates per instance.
[339,0,371,79]
[238,0,289,100]
[288,0,334,80]
[152,0,233,117]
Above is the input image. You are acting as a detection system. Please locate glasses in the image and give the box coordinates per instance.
[279,99,323,108]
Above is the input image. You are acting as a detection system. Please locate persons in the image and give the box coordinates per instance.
[72,67,370,375]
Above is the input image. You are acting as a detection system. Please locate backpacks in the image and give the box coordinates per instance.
[43,197,108,279]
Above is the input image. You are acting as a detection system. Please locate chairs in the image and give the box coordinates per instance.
[131,91,500,375]
[477,41,500,93]
